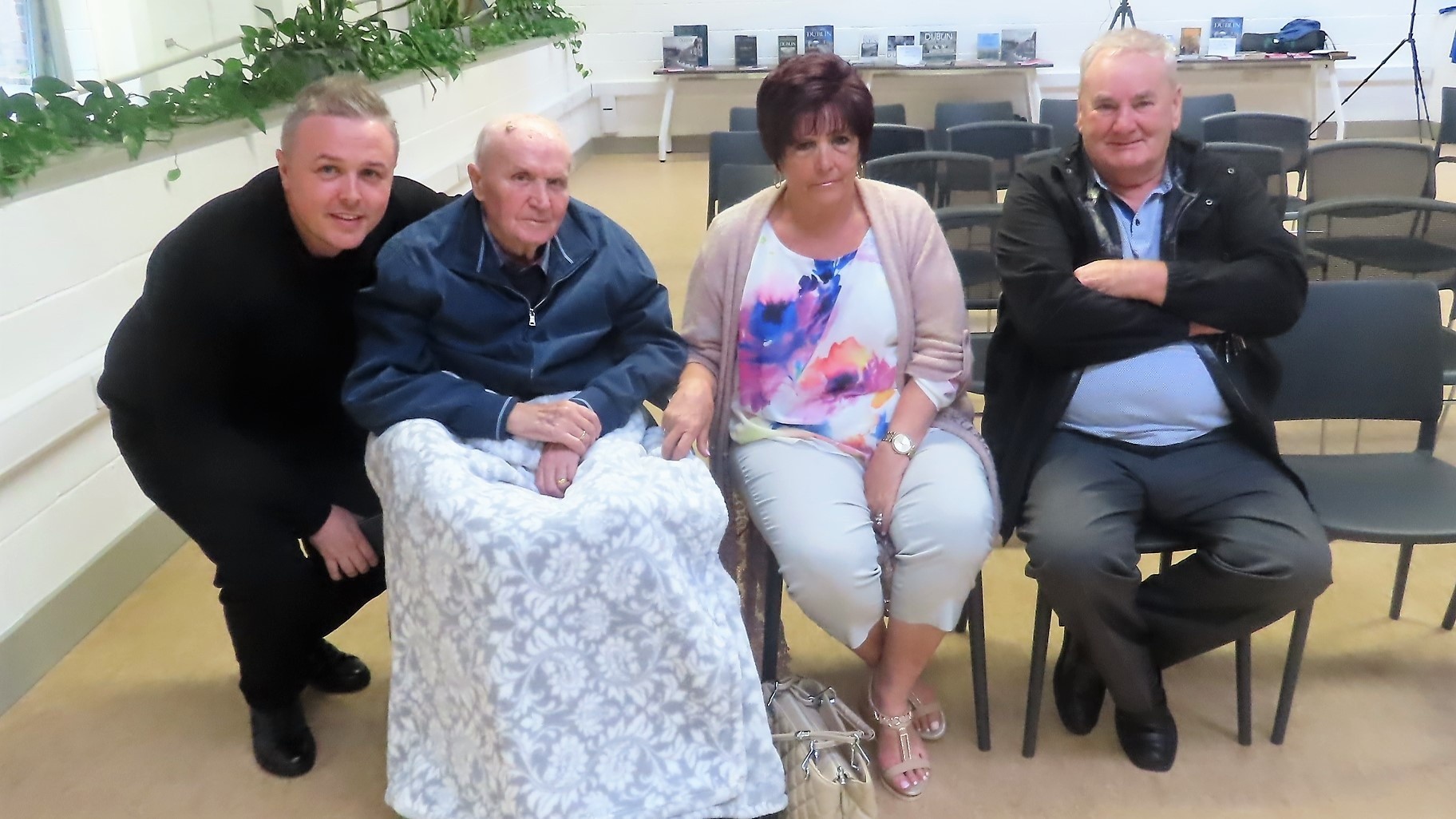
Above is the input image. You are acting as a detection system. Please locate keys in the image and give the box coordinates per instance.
[1219,331,1248,364]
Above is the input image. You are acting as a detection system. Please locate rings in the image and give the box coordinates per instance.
[556,477,567,486]
[874,511,883,526]
[579,429,586,441]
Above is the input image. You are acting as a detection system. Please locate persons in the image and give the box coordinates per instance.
[659,52,1003,800]
[982,27,1333,775]
[342,108,687,502]
[96,70,465,780]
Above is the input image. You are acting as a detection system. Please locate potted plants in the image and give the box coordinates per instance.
[407,0,472,72]
[241,0,382,103]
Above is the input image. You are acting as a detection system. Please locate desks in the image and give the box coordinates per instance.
[1176,55,1356,142]
[655,59,1054,162]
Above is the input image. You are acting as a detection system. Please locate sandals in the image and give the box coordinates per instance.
[860,674,930,801]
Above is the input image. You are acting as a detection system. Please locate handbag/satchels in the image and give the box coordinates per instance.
[762,674,879,818]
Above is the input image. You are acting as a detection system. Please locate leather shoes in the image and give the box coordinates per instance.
[1054,627,1105,735]
[1116,670,1178,772]
[301,637,371,693]
[250,700,315,778]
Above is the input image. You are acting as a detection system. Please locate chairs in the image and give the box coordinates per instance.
[709,87,1456,760]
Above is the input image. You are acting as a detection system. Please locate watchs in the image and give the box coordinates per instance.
[881,429,917,461]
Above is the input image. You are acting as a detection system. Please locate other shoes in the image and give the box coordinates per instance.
[908,690,947,740]
